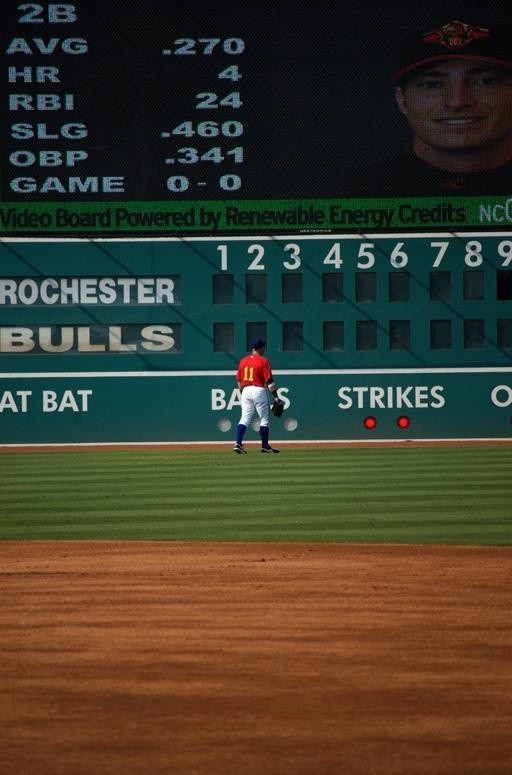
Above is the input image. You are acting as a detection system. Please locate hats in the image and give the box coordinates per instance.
[388,20,511,84]
[251,338,267,350]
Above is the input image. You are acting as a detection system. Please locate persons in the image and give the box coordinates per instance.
[320,12,511,199]
[233,338,279,453]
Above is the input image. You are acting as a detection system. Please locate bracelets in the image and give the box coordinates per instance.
[271,390,277,397]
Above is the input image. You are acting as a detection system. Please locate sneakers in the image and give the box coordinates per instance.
[233,444,247,454]
[261,447,279,453]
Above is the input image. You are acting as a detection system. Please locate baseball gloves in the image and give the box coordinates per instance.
[272,401,284,417]
